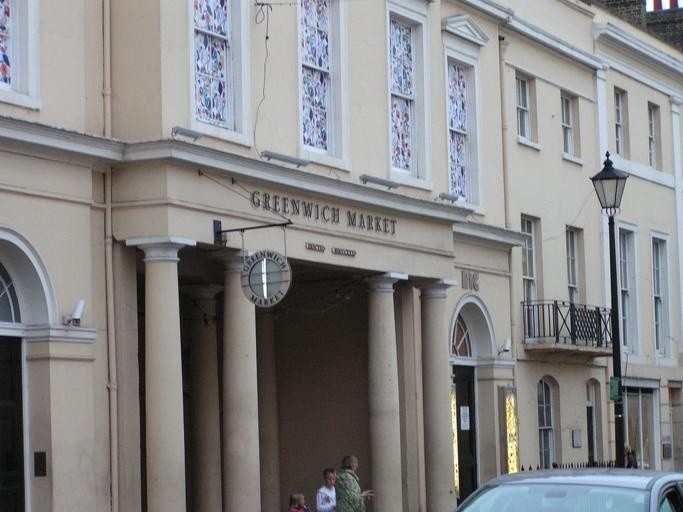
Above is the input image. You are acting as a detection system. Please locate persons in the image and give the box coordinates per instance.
[315,468,336,512]
[290,494,310,512]
[334,455,375,512]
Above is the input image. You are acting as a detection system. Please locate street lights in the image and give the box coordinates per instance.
[589,150,636,466]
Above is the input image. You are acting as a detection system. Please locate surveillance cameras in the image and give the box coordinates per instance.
[71,300,87,326]
[503,338,510,352]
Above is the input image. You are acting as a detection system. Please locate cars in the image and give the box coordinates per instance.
[456,467,682,512]
